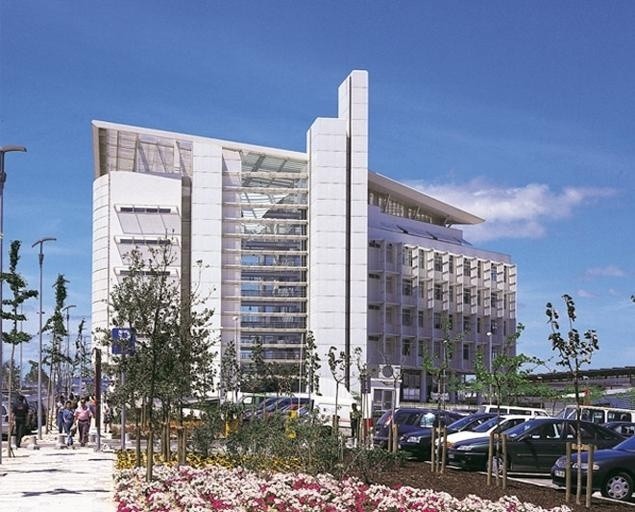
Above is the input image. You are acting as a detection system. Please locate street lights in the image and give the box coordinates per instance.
[31,238,55,440]
[0,145,25,465]
[62,304,75,400]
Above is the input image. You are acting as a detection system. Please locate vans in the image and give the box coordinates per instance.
[481,404,549,417]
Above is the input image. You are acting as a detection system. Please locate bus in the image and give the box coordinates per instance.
[560,404,634,422]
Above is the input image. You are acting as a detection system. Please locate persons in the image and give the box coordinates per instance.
[350,403,361,439]
[56,393,114,447]
[10,394,29,449]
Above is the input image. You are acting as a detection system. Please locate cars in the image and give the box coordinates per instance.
[0,394,47,440]
[373,407,462,455]
[245,397,308,416]
[434,413,633,502]
